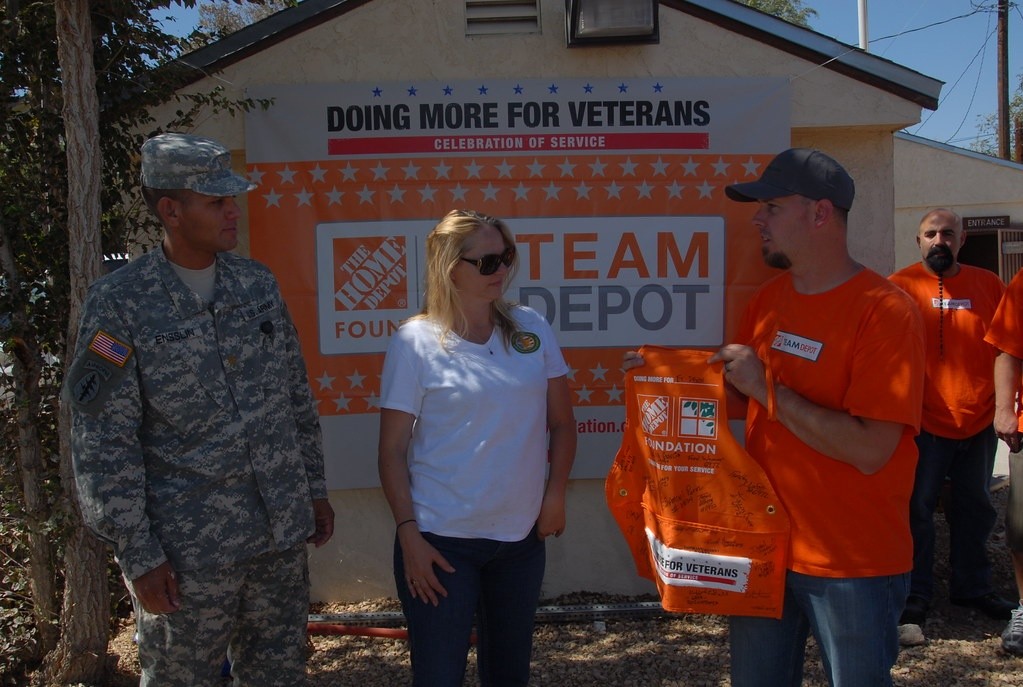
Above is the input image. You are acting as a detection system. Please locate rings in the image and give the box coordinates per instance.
[410,578,417,584]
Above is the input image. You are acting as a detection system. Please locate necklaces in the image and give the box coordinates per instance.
[471,326,496,355]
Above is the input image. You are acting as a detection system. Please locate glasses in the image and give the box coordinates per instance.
[459,246,515,276]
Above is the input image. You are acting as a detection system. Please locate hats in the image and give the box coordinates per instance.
[725,148,855,210]
[140,133,258,196]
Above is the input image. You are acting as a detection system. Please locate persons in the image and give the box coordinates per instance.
[983,268,1023,654]
[622,147,925,687]
[377,208,578,687]
[886,207,1020,626]
[66,132,335,687]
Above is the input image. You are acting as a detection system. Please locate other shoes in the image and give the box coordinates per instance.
[897,594,933,626]
[948,581,1019,620]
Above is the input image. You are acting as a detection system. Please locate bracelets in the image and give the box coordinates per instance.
[397,519,417,533]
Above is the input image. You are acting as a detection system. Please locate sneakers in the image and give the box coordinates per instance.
[1001,599,1023,654]
